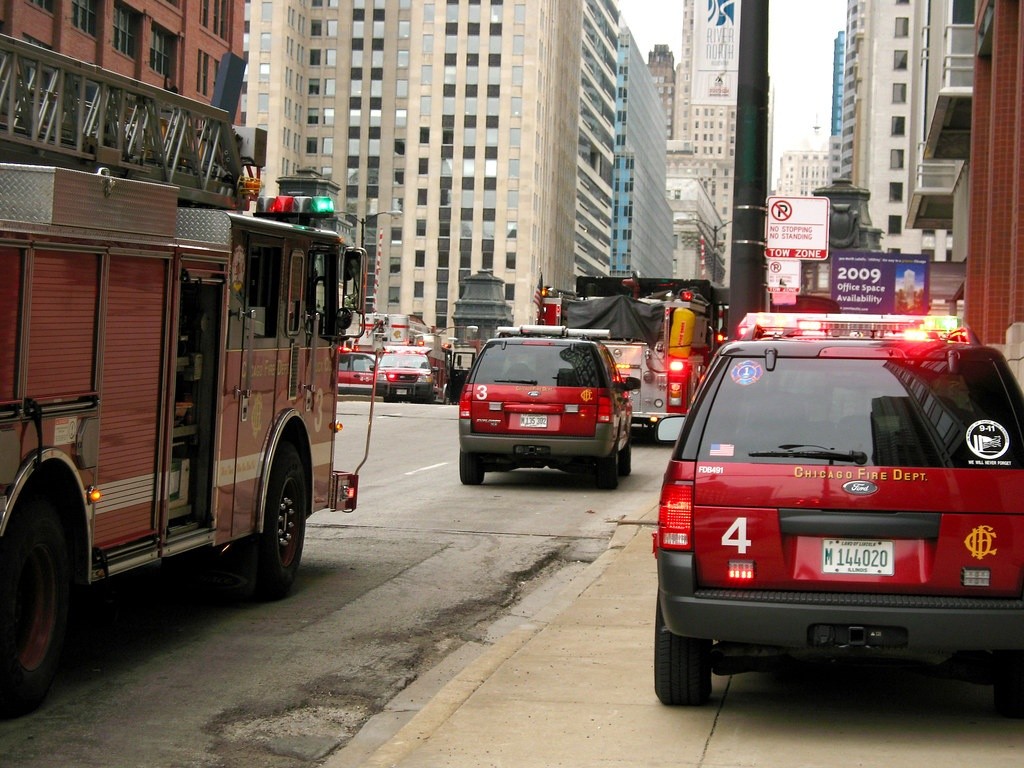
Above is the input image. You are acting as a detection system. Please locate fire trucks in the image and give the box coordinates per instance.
[534,273,729,441]
[0,32,384,727]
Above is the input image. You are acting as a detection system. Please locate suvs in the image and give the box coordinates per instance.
[453,326,643,490]
[652,313,1024,719]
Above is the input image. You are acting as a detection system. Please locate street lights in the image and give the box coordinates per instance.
[335,208,404,334]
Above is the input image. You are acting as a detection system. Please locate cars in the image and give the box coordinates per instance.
[328,312,481,408]
